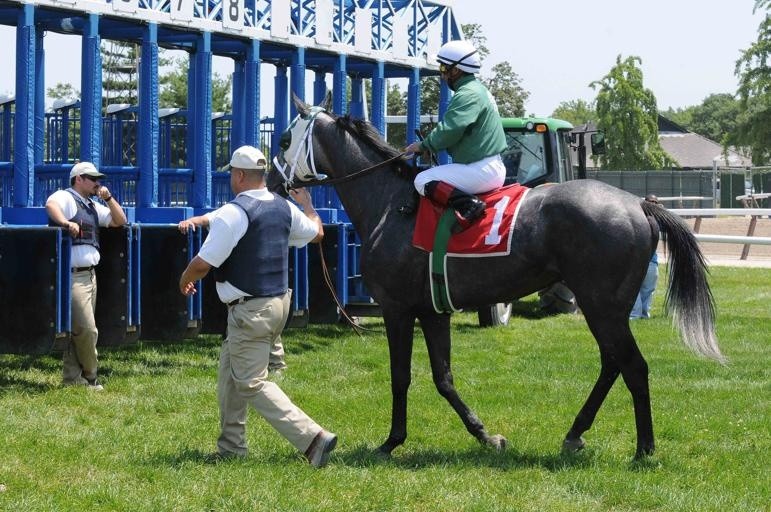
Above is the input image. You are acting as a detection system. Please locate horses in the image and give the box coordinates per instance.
[266,90,729,464]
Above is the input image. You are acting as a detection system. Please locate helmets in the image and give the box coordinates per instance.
[436,40,480,74]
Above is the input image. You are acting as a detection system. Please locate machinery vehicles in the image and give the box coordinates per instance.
[435,119,609,331]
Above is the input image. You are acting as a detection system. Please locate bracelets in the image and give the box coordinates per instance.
[104,194,113,203]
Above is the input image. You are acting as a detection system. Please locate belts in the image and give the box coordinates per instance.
[72,264,96,273]
[229,294,272,306]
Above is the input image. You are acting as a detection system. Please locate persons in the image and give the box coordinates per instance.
[404,40,508,235]
[630,194,664,321]
[46,162,127,391]
[179,144,338,471]
[179,203,287,379]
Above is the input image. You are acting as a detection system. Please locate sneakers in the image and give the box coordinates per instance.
[306,431,337,468]
[63,378,104,392]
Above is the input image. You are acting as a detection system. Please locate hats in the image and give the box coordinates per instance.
[221,146,268,172]
[70,161,105,177]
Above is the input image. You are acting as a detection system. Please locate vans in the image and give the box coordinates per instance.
[716,177,757,204]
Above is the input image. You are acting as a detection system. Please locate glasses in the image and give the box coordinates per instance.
[440,49,477,74]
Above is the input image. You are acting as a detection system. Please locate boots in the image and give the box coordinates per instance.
[424,180,486,233]
